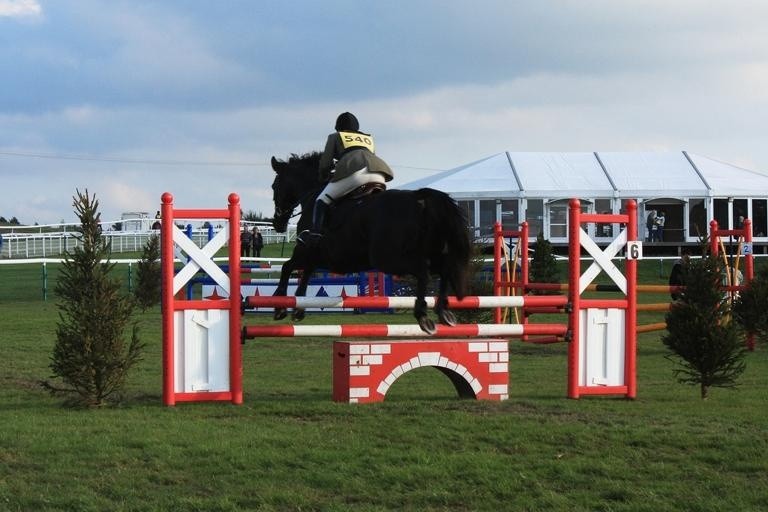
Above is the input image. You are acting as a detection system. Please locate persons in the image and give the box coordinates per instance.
[239,225,252,263]
[668,248,691,302]
[251,227,263,263]
[296,112,393,252]
[656,212,665,243]
[155,211,162,225]
[718,255,743,318]
[646,208,656,242]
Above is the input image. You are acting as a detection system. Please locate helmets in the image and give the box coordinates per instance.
[335,113,358,131]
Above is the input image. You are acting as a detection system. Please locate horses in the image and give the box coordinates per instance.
[270,150,473,335]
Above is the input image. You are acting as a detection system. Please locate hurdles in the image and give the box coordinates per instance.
[493,219,752,347]
[161,192,638,407]
[186,221,395,315]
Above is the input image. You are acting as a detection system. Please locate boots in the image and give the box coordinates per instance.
[295,199,328,253]
